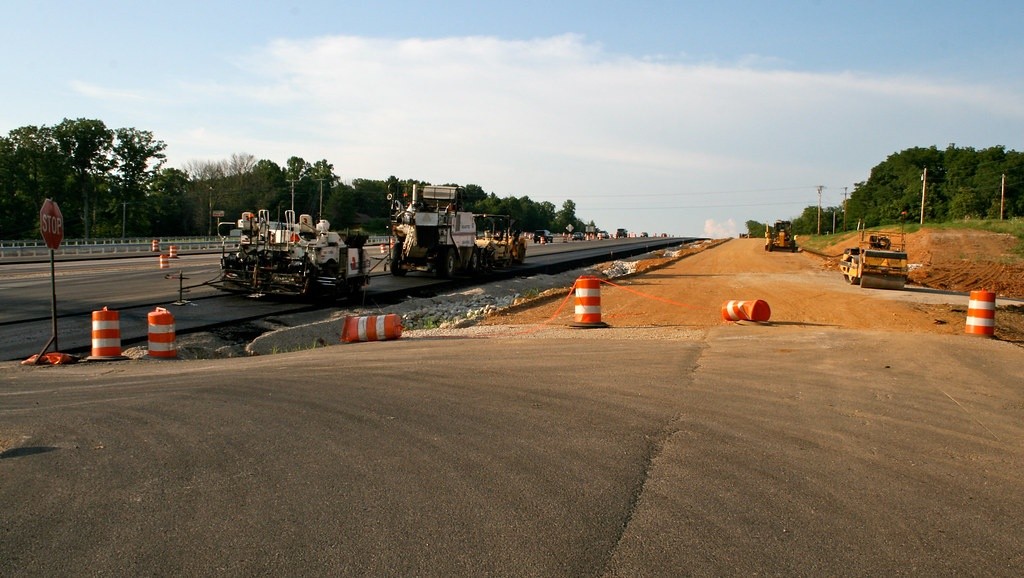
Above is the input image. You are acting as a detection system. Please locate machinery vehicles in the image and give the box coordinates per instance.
[764,220,799,254]
[385,179,528,280]
[837,211,908,290]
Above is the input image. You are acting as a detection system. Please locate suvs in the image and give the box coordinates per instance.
[616,228,628,238]
[533,230,553,243]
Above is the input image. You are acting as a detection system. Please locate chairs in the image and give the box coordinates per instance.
[300,215,311,225]
[495,231,502,240]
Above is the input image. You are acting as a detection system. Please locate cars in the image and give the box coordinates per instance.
[640,232,649,237]
[571,232,584,241]
[597,230,609,239]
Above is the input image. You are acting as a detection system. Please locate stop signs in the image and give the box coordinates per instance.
[40,199,65,249]
